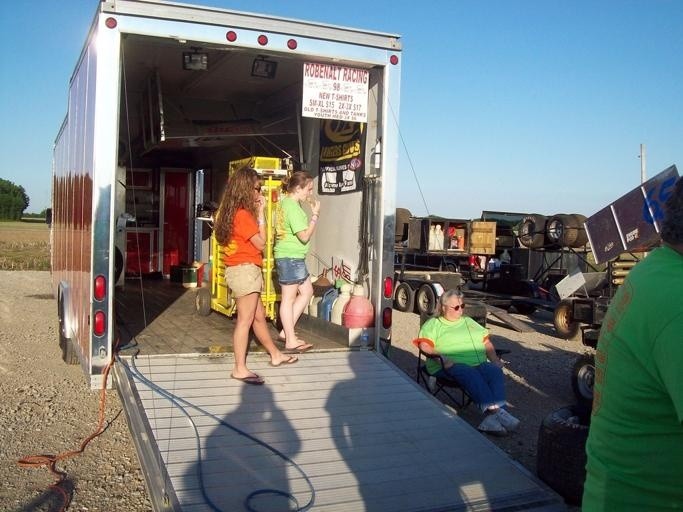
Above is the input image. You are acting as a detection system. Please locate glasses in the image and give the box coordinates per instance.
[253,186,262,191]
[444,303,466,311]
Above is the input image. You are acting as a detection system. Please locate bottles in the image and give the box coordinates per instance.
[359,327,370,352]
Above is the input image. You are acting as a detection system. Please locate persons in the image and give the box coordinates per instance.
[273,168,321,352]
[413,287,521,435]
[579,174,682,512]
[468,249,512,279]
[214,166,298,385]
[428,224,465,252]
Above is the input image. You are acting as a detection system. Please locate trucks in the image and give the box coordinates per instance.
[43,0,402,394]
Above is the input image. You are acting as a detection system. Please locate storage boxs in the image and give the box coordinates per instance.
[170,266,197,288]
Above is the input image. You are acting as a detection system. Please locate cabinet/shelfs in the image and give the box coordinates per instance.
[126,168,160,280]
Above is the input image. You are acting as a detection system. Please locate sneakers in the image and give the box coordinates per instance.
[477,407,521,437]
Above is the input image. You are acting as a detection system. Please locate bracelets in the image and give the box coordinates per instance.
[254,217,266,226]
[309,218,317,225]
[311,213,319,218]
[437,356,448,363]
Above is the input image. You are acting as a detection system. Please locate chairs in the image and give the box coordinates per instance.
[417,312,511,418]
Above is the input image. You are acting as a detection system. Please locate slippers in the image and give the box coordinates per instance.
[277,335,314,352]
[270,355,298,367]
[231,371,264,385]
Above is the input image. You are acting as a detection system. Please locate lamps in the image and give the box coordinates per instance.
[182,47,209,72]
[250,55,278,79]
